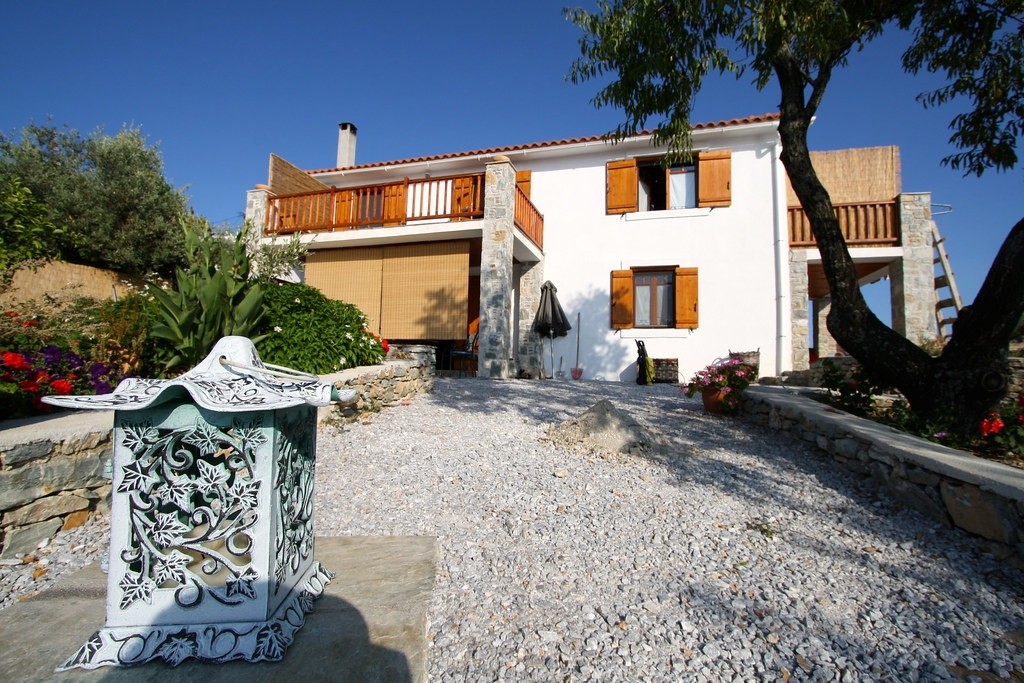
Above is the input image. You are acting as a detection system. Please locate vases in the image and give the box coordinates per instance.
[702,390,732,417]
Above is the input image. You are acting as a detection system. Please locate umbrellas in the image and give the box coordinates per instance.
[531,280,572,379]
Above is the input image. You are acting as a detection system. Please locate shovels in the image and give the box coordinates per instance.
[569,311,584,380]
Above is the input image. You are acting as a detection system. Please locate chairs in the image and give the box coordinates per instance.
[449,330,479,378]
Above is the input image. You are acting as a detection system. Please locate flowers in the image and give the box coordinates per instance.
[680,360,758,411]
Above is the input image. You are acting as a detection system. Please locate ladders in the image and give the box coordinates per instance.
[929,219,965,344]
